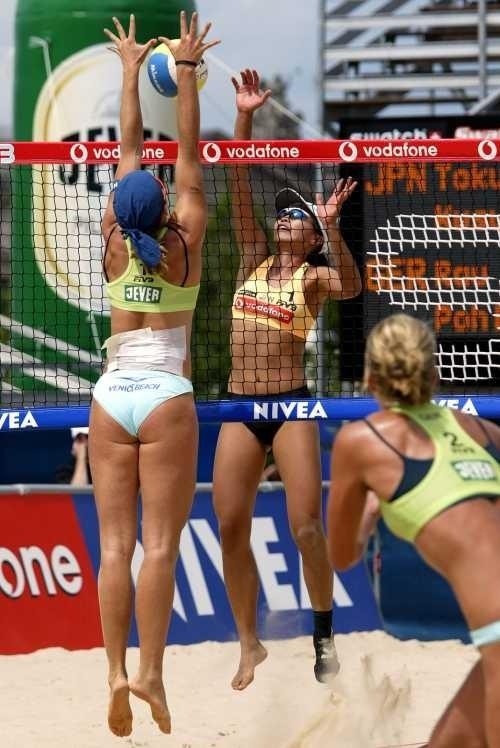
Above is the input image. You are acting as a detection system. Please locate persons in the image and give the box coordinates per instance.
[212,67,367,690]
[54,427,94,486]
[325,311,499,747]
[87,8,219,734]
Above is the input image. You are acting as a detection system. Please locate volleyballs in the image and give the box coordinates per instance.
[147,38,208,99]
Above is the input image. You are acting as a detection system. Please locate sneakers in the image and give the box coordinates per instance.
[313,628,339,682]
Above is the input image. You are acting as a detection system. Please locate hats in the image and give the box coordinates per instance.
[274,189,341,254]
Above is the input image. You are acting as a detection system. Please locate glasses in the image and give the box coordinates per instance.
[277,208,311,219]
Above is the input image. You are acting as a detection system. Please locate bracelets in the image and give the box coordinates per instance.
[175,59,198,66]
